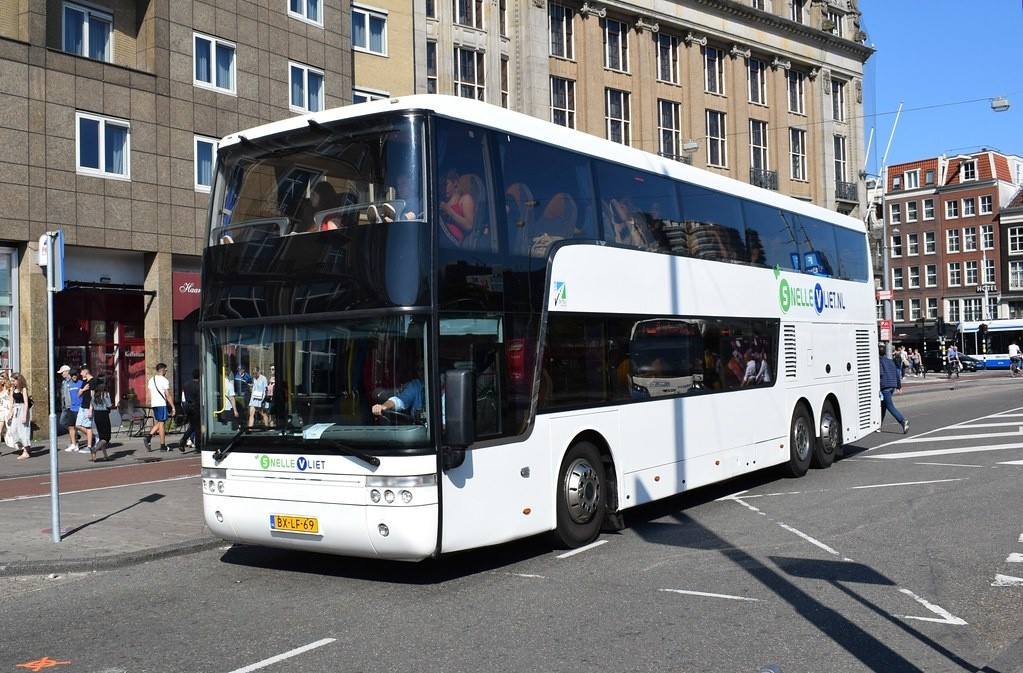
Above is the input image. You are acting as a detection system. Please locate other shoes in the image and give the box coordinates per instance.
[160,445,173,451]
[64,434,113,461]
[187,439,196,448]
[382,203,396,220]
[144,435,151,450]
[17,452,30,459]
[367,205,384,223]
[179,438,186,452]
[902,420,910,434]
[195,447,201,453]
[874,428,881,433]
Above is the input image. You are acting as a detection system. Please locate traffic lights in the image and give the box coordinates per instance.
[915,318,924,336]
[982,325,988,335]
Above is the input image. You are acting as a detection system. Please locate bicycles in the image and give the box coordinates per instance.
[1010,356,1023,378]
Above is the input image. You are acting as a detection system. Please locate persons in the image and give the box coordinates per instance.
[892,344,923,380]
[1007,341,1022,374]
[371,353,445,430]
[56,364,100,453]
[740,352,770,386]
[876,346,910,434]
[0,372,33,460]
[90,374,113,462]
[305,181,343,231]
[143,363,176,451]
[947,345,959,370]
[225,363,276,430]
[178,369,201,453]
[366,167,475,249]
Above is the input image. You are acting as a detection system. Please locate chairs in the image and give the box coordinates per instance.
[168,406,187,433]
[465,173,616,252]
[117,399,155,437]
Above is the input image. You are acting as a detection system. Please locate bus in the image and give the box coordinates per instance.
[955,319,1023,369]
[195,95,882,563]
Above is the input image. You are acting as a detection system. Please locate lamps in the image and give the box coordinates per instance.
[992,97,1010,114]
[684,139,698,153]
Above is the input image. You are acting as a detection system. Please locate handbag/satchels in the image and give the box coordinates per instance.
[252,390,262,399]
[904,360,910,367]
[166,401,172,414]
[958,362,963,370]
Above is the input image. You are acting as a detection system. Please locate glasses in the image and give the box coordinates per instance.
[270,369,274,370]
[417,367,424,372]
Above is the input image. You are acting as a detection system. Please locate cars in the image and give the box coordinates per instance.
[923,349,977,372]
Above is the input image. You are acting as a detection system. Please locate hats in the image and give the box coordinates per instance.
[71,370,79,376]
[76,363,90,370]
[237,364,245,371]
[57,365,71,373]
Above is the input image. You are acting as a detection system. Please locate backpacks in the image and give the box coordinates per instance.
[28,396,34,409]
[893,352,901,366]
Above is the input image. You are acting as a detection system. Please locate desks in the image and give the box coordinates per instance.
[133,405,153,437]
